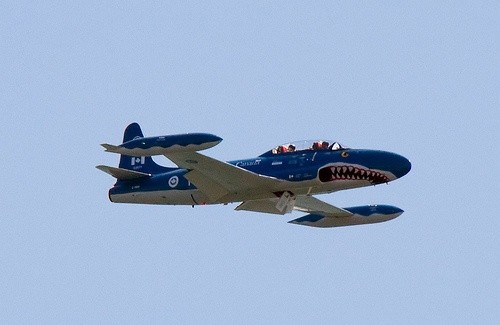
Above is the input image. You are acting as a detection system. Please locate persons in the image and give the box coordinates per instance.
[321,141,329,149]
[286,145,296,152]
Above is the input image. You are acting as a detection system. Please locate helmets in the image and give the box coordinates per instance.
[289,144,295,149]
[322,142,329,147]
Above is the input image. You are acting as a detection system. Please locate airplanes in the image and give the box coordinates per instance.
[96,122,412,228]
